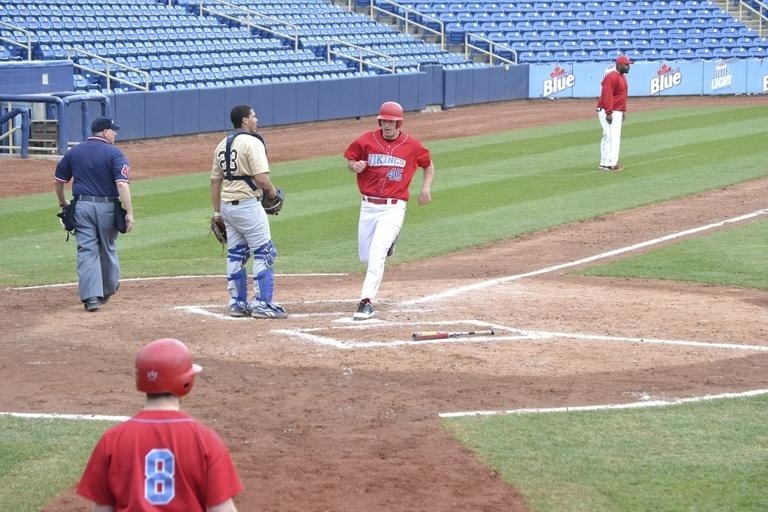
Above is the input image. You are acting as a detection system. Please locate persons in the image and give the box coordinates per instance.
[72,335,243,511]
[593,56,634,172]
[52,116,135,313]
[344,99,436,322]
[210,103,288,321]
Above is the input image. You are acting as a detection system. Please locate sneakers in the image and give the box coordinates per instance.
[85,300,99,310]
[231,301,287,318]
[353,298,375,318]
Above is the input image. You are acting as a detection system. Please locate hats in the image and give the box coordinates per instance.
[92,118,120,131]
[616,57,633,64]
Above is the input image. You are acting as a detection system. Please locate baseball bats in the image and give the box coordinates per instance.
[413,329,494,340]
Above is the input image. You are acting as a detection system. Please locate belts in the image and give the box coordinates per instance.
[77,196,113,202]
[363,196,397,204]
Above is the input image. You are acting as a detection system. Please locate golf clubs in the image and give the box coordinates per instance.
[211,217,227,244]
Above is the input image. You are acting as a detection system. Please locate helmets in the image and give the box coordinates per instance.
[136,338,202,396]
[377,102,404,127]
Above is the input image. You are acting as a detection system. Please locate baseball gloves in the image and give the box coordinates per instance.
[261,188,282,214]
